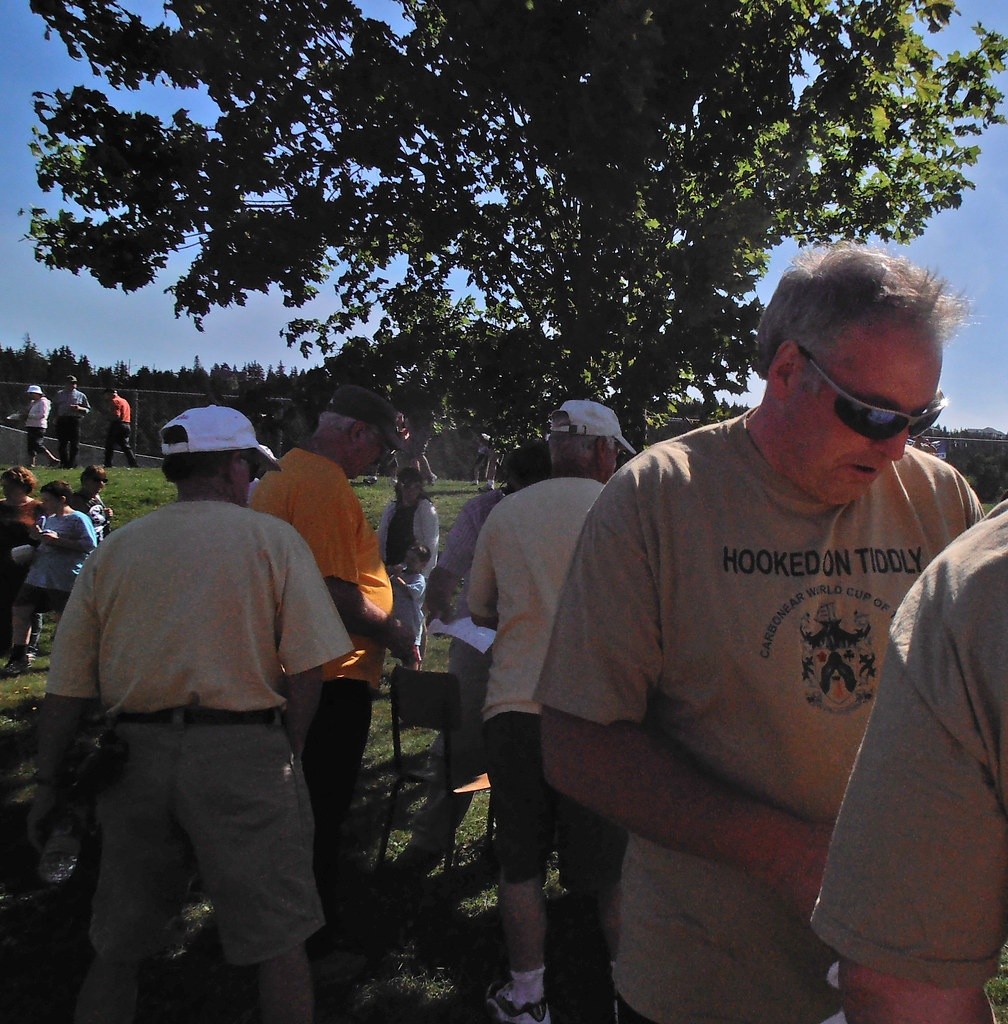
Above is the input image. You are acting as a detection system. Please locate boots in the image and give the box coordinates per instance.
[26,456,36,470]
[42,448,61,468]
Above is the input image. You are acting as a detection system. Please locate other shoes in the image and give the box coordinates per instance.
[478,483,494,491]
[389,478,397,487]
[472,480,479,485]
[426,473,437,486]
[367,475,377,484]
[0,645,29,677]
[28,644,38,653]
[381,847,431,887]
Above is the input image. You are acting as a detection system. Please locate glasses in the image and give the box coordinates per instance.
[87,476,108,483]
[798,345,945,442]
[365,423,394,467]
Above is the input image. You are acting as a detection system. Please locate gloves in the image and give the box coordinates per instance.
[27,775,62,855]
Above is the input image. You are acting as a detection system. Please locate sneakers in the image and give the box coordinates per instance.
[484,980,552,1024]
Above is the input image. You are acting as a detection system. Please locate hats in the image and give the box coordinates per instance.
[64,375,77,382]
[26,385,44,394]
[158,405,283,472]
[102,388,119,395]
[324,385,411,456]
[549,400,637,455]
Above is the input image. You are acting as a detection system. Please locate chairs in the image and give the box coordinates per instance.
[379,665,494,878]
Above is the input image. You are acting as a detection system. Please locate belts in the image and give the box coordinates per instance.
[116,707,285,727]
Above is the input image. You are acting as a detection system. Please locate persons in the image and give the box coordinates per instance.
[251,383,422,979]
[377,468,439,659]
[0,465,42,656]
[363,401,438,486]
[467,399,637,1024]
[382,544,431,682]
[5,479,98,673]
[809,500,1008,1024]
[100,387,139,468]
[470,444,504,492]
[17,385,60,469]
[48,375,91,470]
[25,404,355,1023]
[374,438,554,912]
[55,465,113,623]
[532,239,987,1024]
